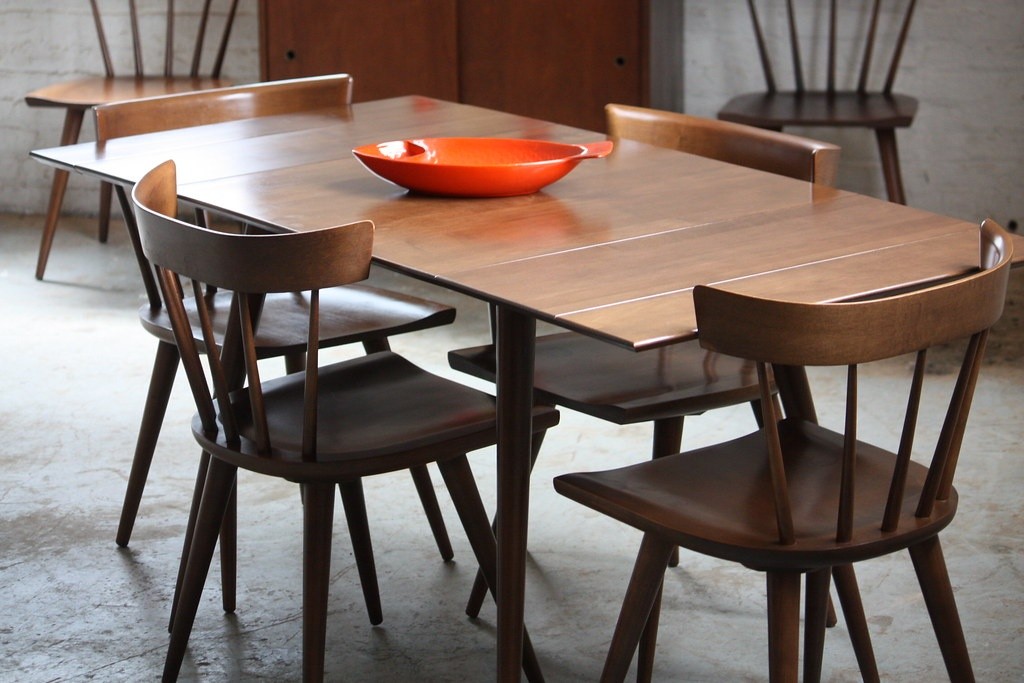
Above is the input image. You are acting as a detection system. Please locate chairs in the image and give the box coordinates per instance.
[553,218,1014,683]
[93,73,457,615]
[25,0,239,281]
[446,104,842,683]
[131,160,560,683]
[716,0,918,207]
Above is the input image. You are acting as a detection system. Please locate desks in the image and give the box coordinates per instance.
[30,94,1024,683]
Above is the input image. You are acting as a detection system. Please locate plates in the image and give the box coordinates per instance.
[351,136,613,198]
